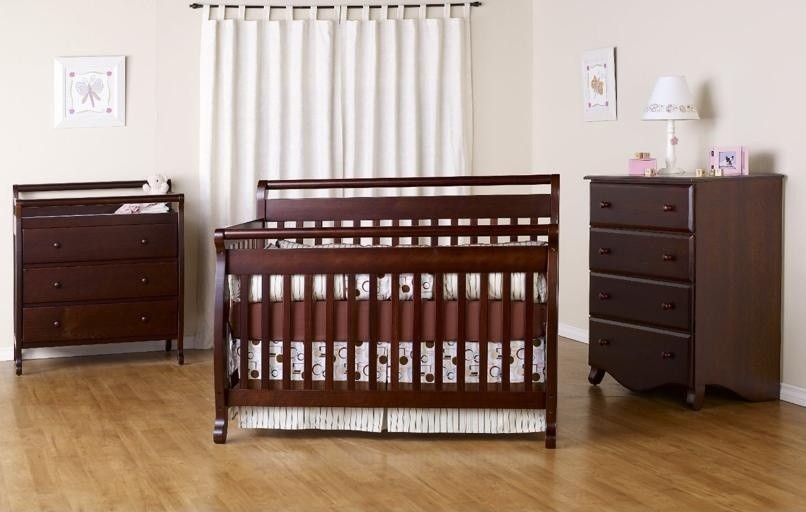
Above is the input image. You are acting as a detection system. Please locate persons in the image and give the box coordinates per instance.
[720,155,734,166]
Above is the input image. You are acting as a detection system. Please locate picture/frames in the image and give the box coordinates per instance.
[706,145,750,178]
[581,47,618,123]
[53,53,127,130]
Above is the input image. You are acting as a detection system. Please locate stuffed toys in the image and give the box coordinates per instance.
[142,173,169,207]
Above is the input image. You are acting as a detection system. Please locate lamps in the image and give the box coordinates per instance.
[642,72,703,176]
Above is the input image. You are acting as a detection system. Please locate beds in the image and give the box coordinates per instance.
[210,172,563,451]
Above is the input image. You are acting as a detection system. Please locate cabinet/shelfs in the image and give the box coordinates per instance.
[583,172,782,413]
[10,178,191,382]
